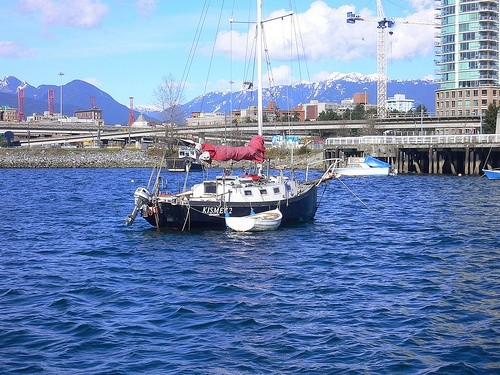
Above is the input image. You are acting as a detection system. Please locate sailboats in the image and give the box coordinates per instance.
[123,0,341,232]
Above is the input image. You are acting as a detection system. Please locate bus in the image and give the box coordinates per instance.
[105,139,165,152]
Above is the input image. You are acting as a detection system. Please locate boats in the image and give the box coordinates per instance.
[482,167,500,180]
[167,149,205,172]
[330,155,397,176]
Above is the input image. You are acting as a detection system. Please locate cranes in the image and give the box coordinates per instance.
[346,1,435,118]
